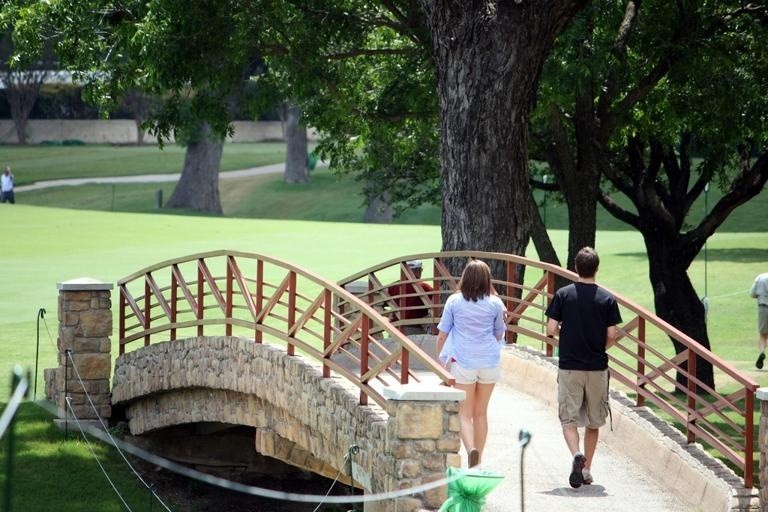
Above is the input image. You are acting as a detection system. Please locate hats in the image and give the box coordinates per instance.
[406,259,422,269]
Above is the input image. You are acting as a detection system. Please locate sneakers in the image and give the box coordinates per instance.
[569,452,587,488]
[469,448,479,467]
[582,471,593,485]
[756,353,765,369]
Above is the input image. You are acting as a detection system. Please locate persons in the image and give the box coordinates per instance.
[388,260,434,336]
[0,165,16,204]
[544,246,623,488]
[750,273,768,369]
[438,259,504,468]
